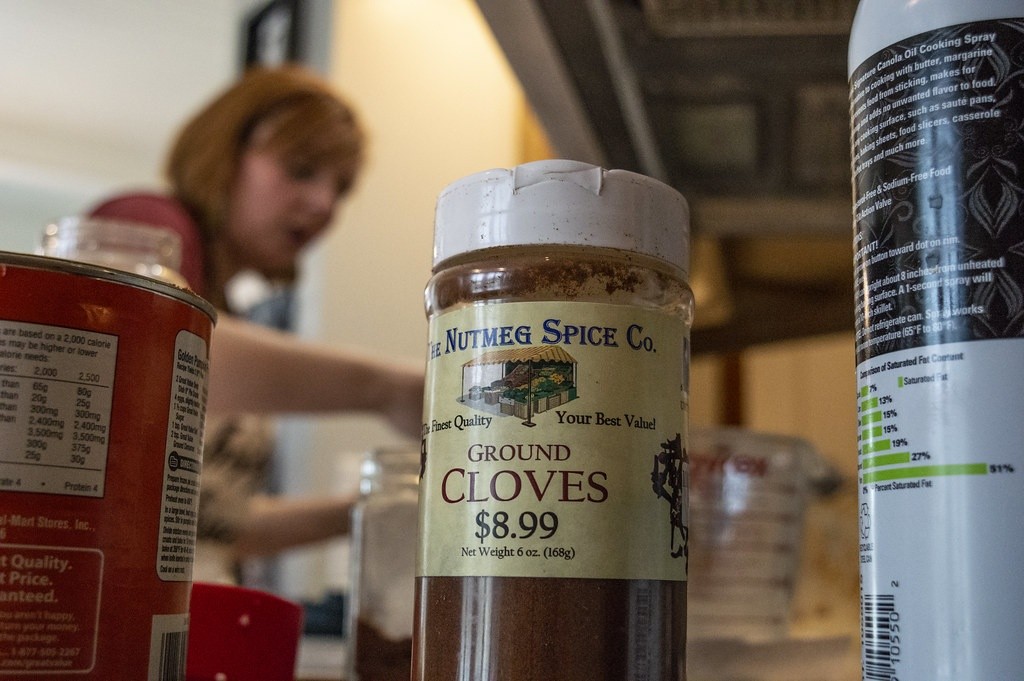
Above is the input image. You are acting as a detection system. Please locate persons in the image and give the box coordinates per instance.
[79,61,425,555]
[190,413,272,588]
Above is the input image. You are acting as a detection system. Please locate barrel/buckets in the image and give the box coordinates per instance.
[688,424,811,632]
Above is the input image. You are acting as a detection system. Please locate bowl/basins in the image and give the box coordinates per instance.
[186,579,303,681]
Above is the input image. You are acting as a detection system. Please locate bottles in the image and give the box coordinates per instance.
[849,0,1024,681]
[343,449,417,673]
[36,214,198,297]
